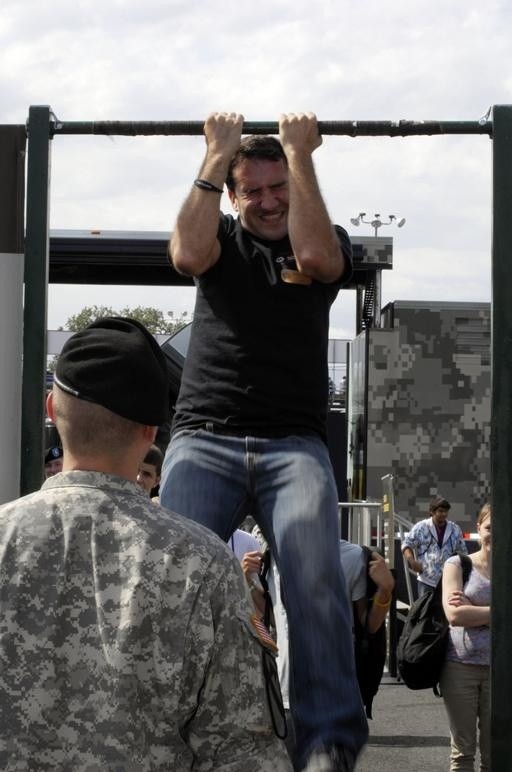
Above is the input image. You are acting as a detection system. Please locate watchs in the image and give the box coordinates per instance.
[249,578,257,592]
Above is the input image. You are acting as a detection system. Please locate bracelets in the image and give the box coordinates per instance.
[374,593,391,607]
[191,178,225,196]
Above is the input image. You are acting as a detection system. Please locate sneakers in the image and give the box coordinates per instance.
[299,742,353,772]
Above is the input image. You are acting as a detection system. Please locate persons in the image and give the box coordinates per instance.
[137,444,164,493]
[436,500,494,772]
[42,447,63,479]
[226,528,263,592]
[0,315,296,772]
[400,497,468,598]
[150,109,369,771]
[241,539,398,772]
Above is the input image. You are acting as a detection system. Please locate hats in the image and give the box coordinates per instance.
[53,317,169,426]
[44,447,63,464]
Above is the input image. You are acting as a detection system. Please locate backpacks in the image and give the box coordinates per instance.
[397,551,472,698]
[258,544,386,719]
